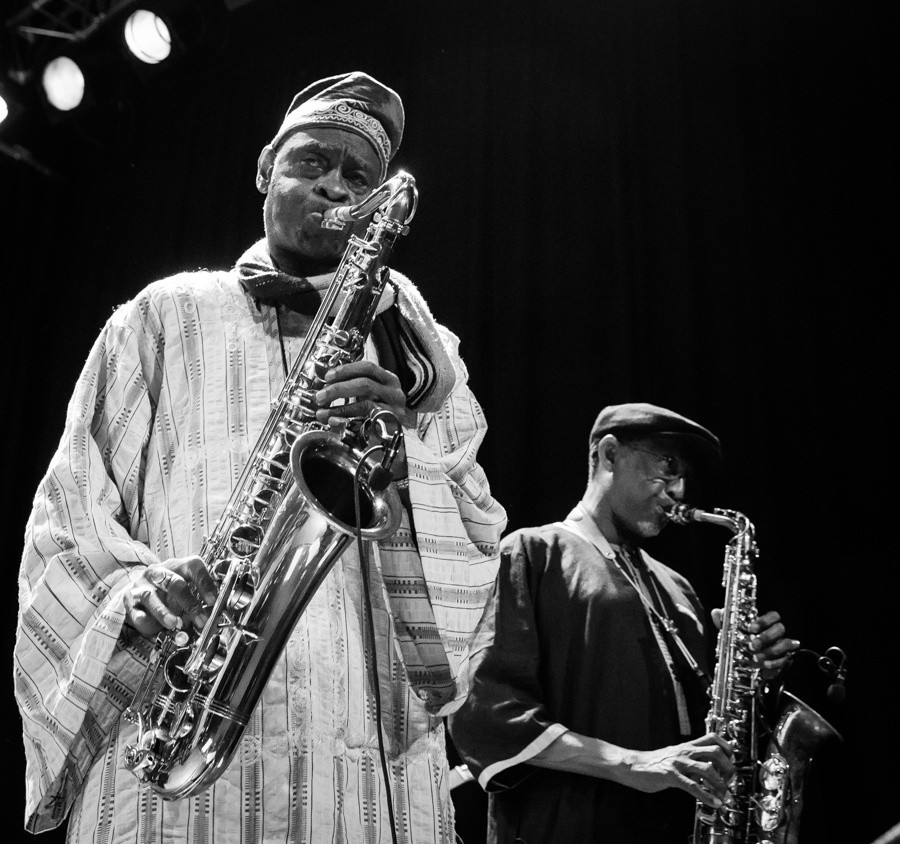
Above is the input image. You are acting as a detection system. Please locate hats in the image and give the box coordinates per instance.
[274,72,405,189]
[590,401,720,461]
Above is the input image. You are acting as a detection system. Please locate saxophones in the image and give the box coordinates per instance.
[660,497,849,844]
[125,163,426,803]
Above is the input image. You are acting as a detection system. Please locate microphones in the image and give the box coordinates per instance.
[368,431,403,491]
[827,667,846,703]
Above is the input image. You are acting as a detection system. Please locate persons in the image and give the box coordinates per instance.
[12,71,508,843]
[449,403,799,844]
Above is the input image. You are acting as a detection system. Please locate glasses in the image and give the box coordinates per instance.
[622,444,695,483]
